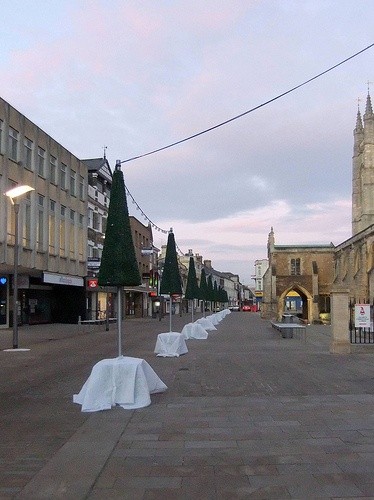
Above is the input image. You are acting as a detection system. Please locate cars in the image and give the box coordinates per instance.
[227,305,240,311]
[243,306,251,311]
[213,306,222,312]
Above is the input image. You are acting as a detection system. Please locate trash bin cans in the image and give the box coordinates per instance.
[281,314,294,338]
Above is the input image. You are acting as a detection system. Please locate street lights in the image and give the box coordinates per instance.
[2,184,36,351]
